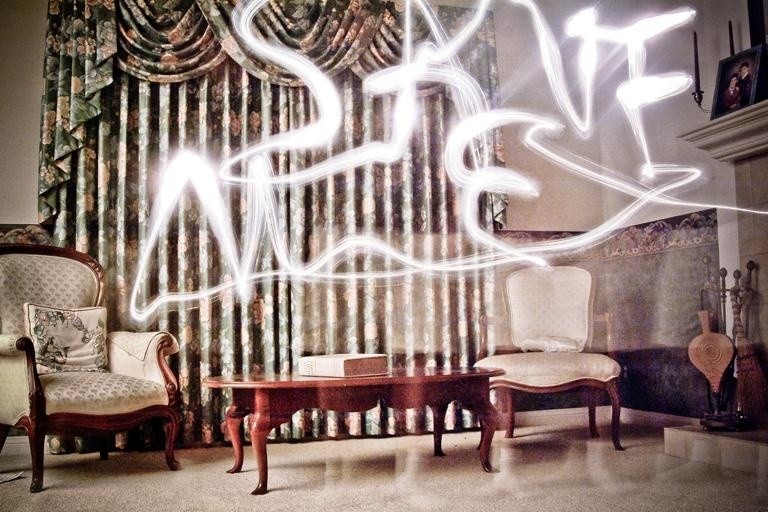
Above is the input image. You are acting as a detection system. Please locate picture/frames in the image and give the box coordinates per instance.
[710,44,762,120]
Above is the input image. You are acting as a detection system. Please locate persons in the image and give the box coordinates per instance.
[715,73,741,115]
[737,61,752,108]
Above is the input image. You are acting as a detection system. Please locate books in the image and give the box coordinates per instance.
[296,349,388,378]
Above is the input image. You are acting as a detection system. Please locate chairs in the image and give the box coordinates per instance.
[0,243,624,495]
